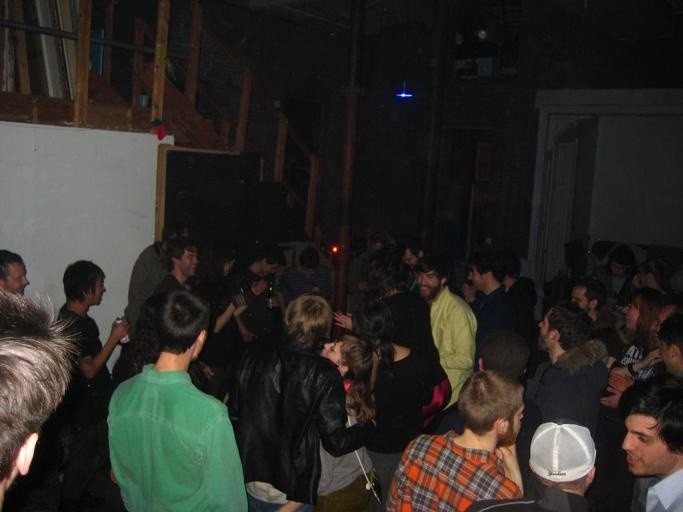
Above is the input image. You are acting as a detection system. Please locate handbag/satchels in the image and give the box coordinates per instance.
[359,469,383,505]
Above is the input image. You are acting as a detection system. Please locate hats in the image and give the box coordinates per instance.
[530,417,597,483]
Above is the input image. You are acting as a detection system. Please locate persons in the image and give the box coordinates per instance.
[123,236,200,379]
[124,213,196,344]
[1,249,29,295]
[1,290,85,512]
[189,216,682,512]
[106,291,249,512]
[57,261,130,512]
